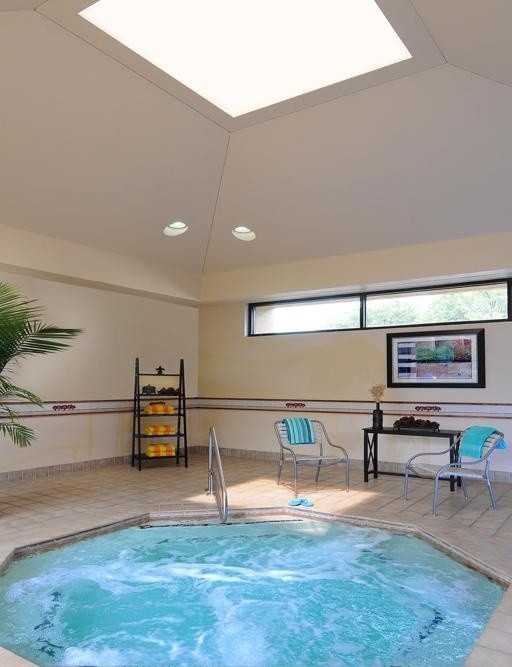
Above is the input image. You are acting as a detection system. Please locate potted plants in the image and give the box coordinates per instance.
[368,384,386,429]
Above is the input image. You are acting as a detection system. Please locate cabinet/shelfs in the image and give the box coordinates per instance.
[131,357,189,471]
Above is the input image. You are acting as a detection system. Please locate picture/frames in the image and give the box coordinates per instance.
[387,329,485,388]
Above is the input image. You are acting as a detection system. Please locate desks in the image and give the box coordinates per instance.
[361,426,462,492]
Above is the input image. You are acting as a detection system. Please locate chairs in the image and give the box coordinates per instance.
[273,417,349,498]
[403,425,505,520]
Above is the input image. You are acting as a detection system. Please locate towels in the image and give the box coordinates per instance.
[282,418,317,444]
[458,425,507,460]
